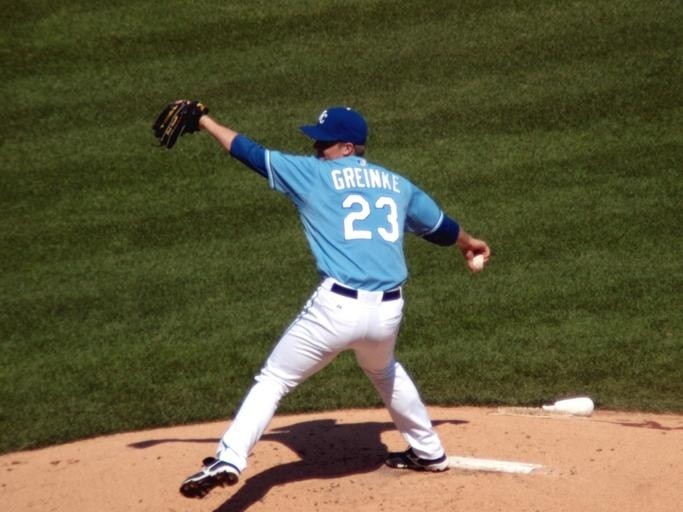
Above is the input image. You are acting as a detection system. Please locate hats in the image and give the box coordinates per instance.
[298,106,368,149]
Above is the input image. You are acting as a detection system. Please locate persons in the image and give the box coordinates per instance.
[151,99,492,498]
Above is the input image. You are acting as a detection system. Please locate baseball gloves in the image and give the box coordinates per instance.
[154,100,208,148]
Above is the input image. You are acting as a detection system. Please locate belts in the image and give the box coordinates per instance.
[331,283,400,303]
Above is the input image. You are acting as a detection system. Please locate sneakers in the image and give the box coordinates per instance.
[384,445,449,473]
[180,455,240,500]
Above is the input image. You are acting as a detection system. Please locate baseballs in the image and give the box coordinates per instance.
[470,254,485,272]
